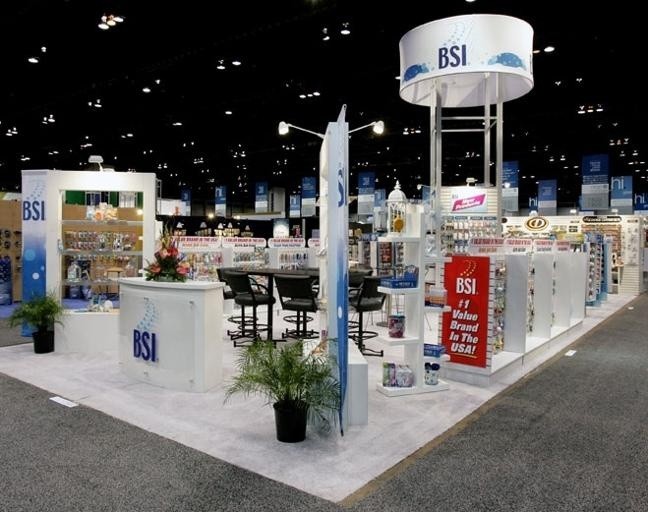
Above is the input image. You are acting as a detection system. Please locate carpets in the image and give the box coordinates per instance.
[1,297,648,512]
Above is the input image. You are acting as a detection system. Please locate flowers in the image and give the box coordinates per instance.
[143,212,189,283]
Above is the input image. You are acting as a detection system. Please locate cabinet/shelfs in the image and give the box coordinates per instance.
[61,219,143,285]
[350,216,638,397]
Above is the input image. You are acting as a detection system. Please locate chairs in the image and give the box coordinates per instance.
[216,264,393,357]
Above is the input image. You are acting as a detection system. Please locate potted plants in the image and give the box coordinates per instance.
[223,336,341,441]
[6,288,65,353]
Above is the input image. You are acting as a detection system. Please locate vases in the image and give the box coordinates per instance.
[159,272,175,281]
